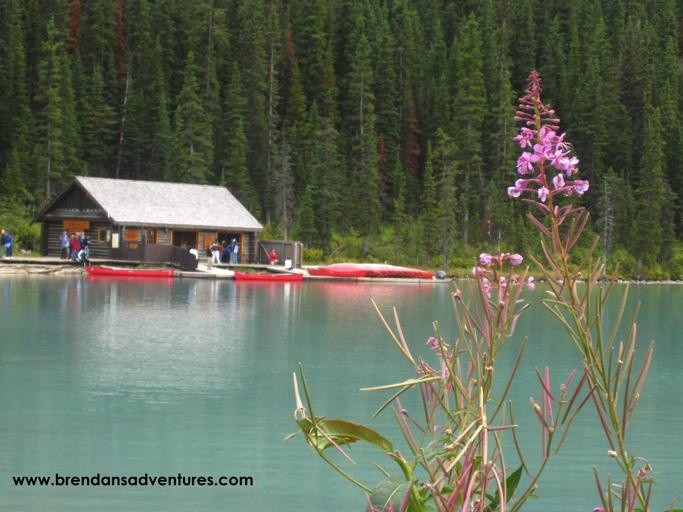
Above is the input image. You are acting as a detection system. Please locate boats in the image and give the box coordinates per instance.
[86,265,175,278]
[307,264,432,279]
[235,271,303,281]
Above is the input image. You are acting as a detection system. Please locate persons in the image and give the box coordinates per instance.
[188,245,198,261]
[59,230,90,267]
[207,236,239,267]
[270,248,278,264]
[284,256,292,271]
[1,229,12,257]
